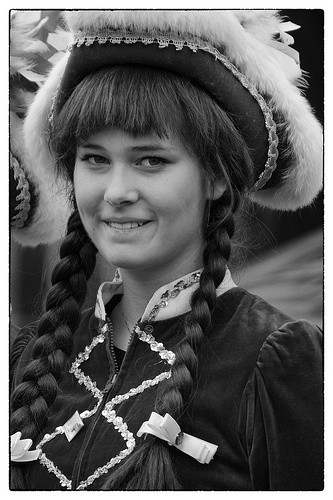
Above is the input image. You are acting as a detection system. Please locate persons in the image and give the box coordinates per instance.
[10,9,324,490]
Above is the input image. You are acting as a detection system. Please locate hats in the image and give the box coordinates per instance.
[23,10,321,212]
[9,9,75,248]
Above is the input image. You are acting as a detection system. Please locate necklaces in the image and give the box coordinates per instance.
[119,299,133,338]
